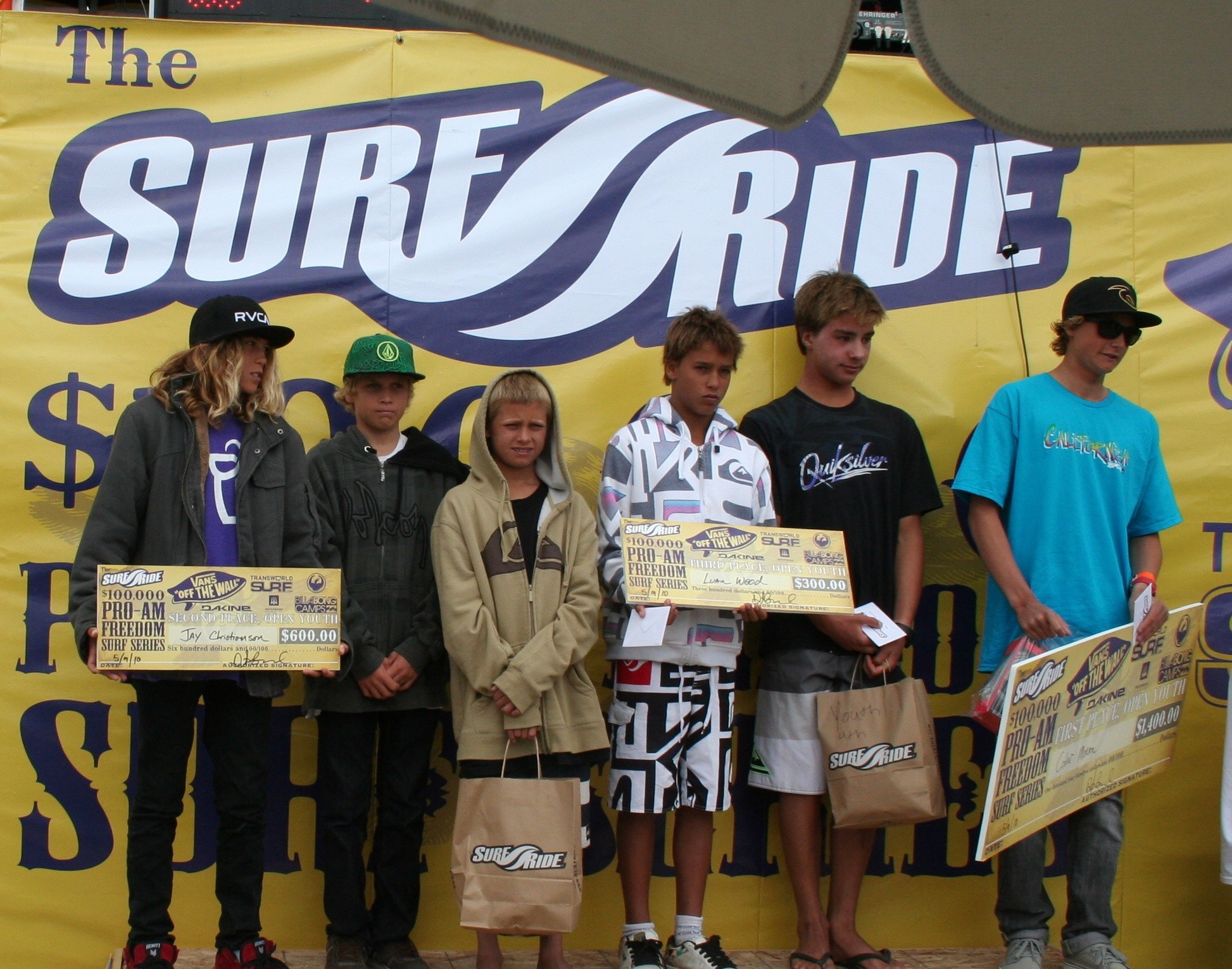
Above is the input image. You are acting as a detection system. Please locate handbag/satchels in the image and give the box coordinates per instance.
[451,731,580,936]
[815,653,948,831]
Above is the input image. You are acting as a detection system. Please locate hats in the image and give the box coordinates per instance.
[343,333,425,380]
[189,295,294,349]
[1062,277,1162,328]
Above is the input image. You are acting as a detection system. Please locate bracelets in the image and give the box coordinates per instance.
[1132,571,1155,582]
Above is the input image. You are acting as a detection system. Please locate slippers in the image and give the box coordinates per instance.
[836,948,891,969]
[790,951,834,969]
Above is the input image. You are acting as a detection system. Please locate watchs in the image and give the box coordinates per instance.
[898,621,915,648]
[1129,577,1157,597]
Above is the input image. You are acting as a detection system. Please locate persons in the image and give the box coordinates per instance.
[739,273,943,969]
[951,276,1182,969]
[599,306,777,969]
[71,294,353,969]
[304,334,472,969]
[432,369,613,969]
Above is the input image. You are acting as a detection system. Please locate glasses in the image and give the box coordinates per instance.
[1083,316,1142,345]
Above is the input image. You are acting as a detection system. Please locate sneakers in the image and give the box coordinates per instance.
[617,931,666,969]
[368,937,430,969]
[997,937,1045,969]
[1063,941,1128,969]
[663,935,736,969]
[123,932,178,969]
[323,931,365,969]
[215,935,289,969]
